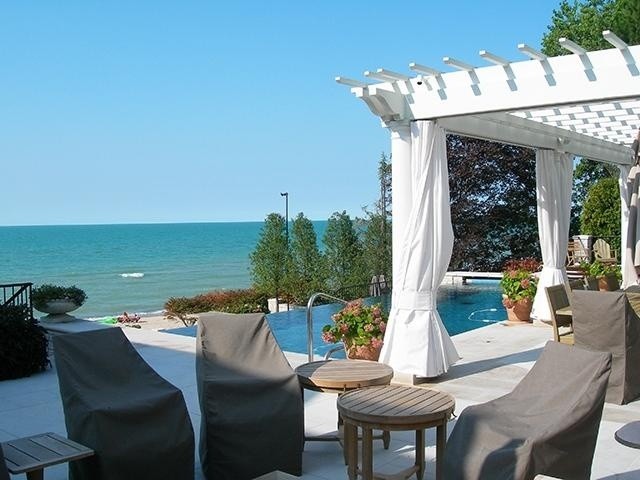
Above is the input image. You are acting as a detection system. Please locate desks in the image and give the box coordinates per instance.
[337,387,456,480]
[295,360,393,450]
[556,293,640,315]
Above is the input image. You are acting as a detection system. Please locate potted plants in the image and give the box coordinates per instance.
[589,260,620,291]
[29,286,86,321]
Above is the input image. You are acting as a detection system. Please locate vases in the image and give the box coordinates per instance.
[503,300,532,321]
[344,337,382,361]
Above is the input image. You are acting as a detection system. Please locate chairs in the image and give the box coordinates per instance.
[54,327,194,480]
[585,275,598,292]
[568,242,590,266]
[544,284,572,343]
[572,290,640,403]
[455,341,611,480]
[199,313,302,480]
[569,279,585,292]
[593,239,617,267]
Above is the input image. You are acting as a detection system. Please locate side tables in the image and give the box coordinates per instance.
[0,432,95,480]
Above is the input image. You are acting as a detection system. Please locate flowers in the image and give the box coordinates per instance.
[499,268,539,308]
[321,297,389,360]
